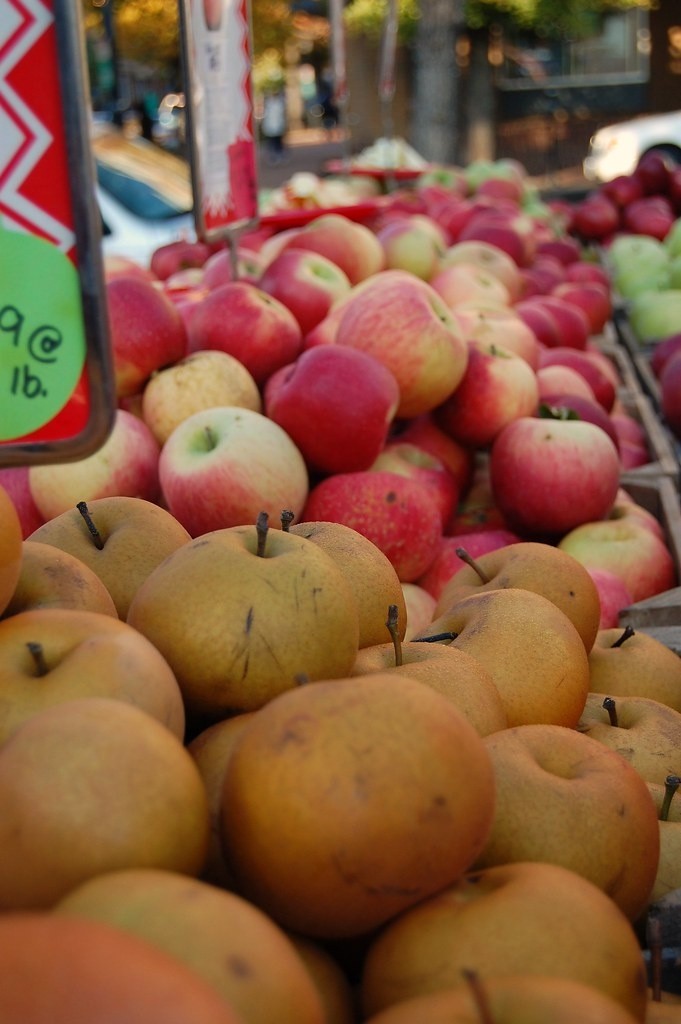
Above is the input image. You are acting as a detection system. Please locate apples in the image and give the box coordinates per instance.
[0,147,681,626]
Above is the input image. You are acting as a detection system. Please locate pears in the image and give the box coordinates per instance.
[0,496,681,1024]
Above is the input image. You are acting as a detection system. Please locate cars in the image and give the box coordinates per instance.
[583,110,681,184]
